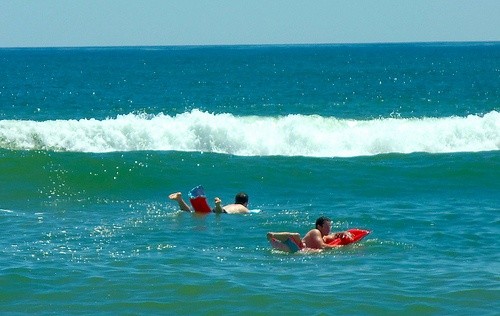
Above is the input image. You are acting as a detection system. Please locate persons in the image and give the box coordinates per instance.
[300,216,354,247]
[169,192,263,213]
